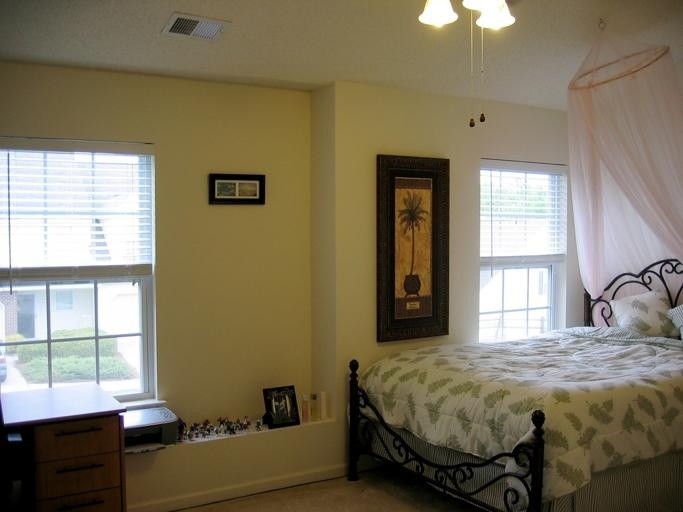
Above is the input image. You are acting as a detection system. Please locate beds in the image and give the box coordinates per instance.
[345,256,681,512]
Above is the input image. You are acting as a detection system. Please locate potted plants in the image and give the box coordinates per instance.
[394,191,430,298]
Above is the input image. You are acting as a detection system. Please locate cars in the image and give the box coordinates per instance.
[0,346,7,384]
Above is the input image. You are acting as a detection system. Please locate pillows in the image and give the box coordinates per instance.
[666,303,682,340]
[608,288,680,339]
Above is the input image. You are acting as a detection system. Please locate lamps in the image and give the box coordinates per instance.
[418,0,514,129]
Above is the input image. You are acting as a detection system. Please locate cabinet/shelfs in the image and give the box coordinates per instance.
[0,382,129,512]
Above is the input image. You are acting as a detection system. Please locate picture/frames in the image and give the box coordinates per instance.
[373,150,451,343]
[208,171,266,207]
[260,384,300,431]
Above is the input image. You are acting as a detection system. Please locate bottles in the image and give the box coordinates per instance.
[301,393,319,422]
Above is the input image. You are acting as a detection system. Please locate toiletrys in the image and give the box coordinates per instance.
[302,394,309,423]
[310,394,319,421]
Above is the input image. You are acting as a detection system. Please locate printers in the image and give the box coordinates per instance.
[119,406,180,454]
